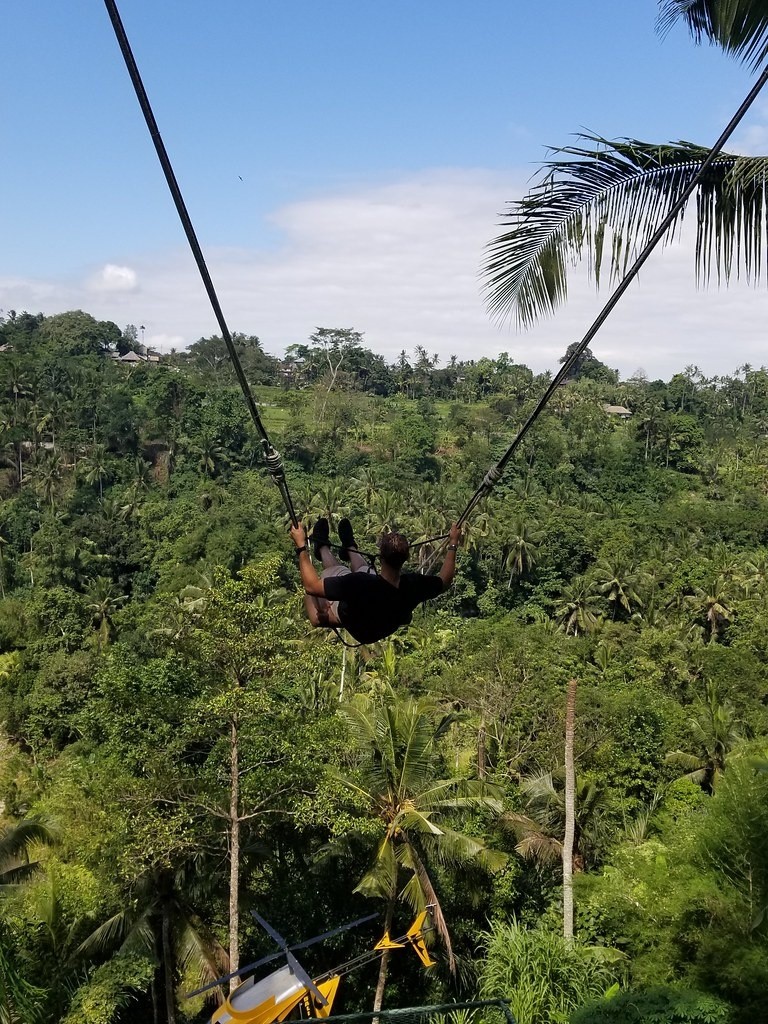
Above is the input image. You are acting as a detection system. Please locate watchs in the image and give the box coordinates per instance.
[294,546,306,555]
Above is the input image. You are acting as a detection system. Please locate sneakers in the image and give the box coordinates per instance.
[338,519,358,562]
[313,518,331,560]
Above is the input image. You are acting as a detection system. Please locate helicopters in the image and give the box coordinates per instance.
[186,903,438,1024]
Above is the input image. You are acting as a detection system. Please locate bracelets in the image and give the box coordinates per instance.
[447,545,458,551]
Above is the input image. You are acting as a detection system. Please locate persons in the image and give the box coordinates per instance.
[294,514,463,644]
